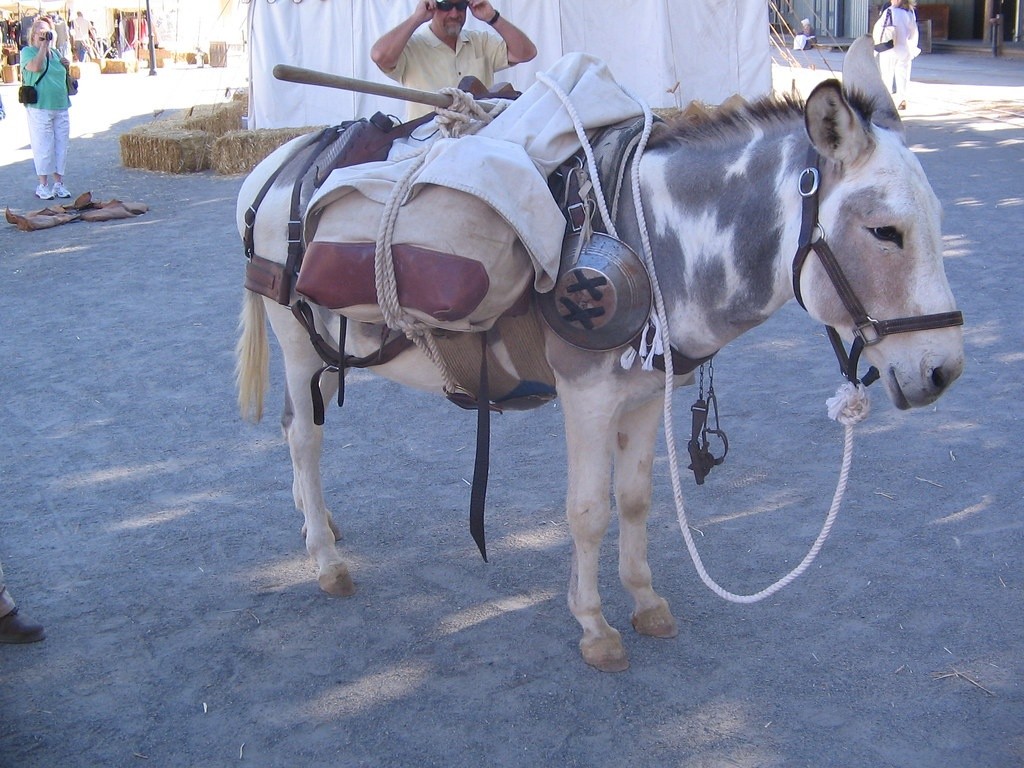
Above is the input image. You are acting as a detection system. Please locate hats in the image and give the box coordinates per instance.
[801,18,810,26]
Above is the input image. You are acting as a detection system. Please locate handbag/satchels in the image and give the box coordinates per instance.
[19,86,37,104]
[874,9,895,53]
[294,183,533,333]
[52,48,78,95]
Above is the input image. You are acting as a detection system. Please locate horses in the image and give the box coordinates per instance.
[234,34,964,673]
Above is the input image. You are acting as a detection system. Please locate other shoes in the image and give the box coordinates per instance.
[0,607,47,644]
[898,100,906,110]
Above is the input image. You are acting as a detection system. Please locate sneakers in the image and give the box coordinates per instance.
[36,182,71,200]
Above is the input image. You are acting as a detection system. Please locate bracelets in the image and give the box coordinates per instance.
[487,10,500,25]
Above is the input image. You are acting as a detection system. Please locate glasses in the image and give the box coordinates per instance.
[436,0,469,11]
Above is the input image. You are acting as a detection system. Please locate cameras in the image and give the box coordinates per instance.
[45,32,53,40]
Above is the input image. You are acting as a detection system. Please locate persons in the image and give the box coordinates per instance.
[0,564,47,644]
[792,18,817,51]
[370,0,537,134]
[21,18,71,200]
[873,0,922,111]
[72,11,97,64]
